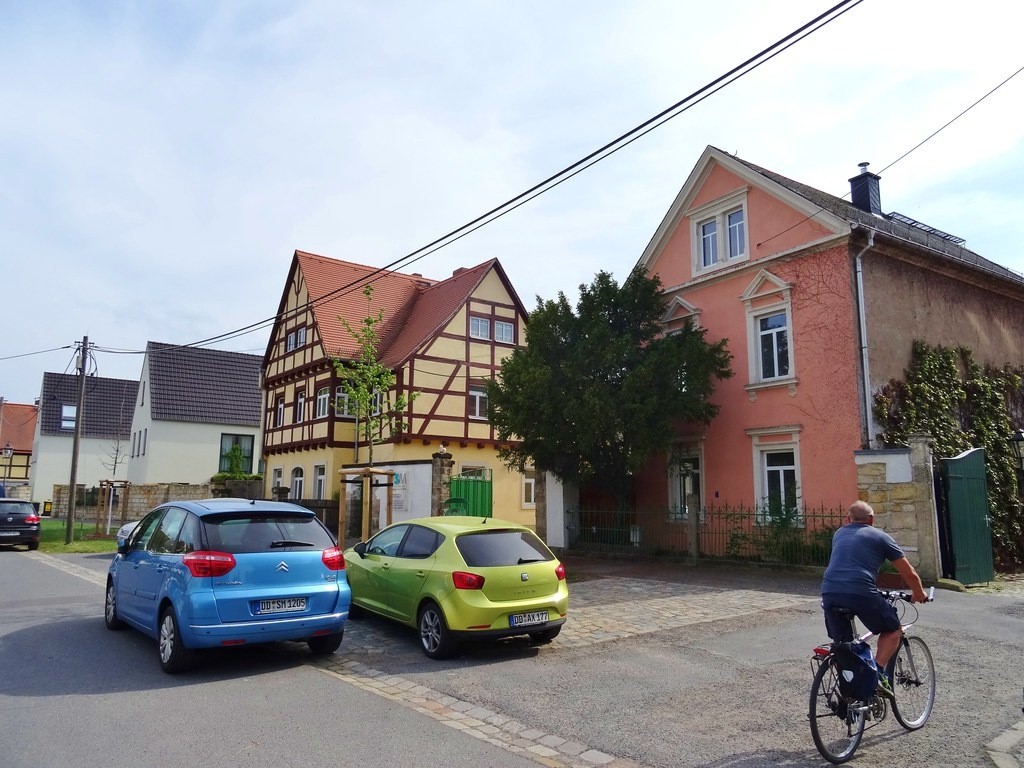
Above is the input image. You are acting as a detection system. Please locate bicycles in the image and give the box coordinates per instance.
[807,586,935,764]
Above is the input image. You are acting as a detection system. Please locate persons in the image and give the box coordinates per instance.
[820,498,928,699]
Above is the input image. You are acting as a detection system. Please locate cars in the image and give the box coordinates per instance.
[105,497,351,674]
[343,516,569,660]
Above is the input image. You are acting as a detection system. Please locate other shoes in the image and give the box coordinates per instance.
[877,671,894,698]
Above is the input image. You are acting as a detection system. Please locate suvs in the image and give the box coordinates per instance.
[0,498,41,550]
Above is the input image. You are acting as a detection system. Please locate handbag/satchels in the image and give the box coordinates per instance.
[835,640,878,701]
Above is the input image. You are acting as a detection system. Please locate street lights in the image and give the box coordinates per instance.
[2,442,14,498]
